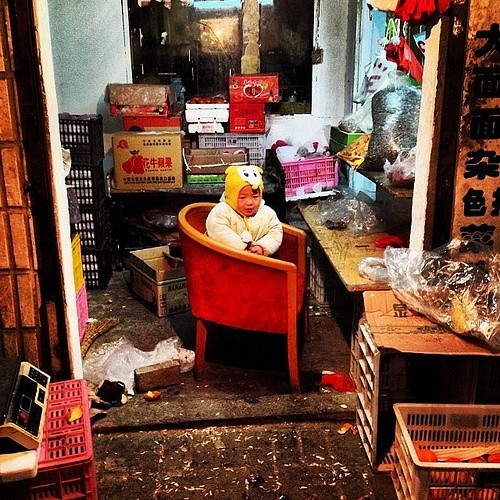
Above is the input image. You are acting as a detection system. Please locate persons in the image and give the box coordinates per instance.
[205,165,284,260]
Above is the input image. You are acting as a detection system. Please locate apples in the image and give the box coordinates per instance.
[188,96,224,104]
[415,448,500,500]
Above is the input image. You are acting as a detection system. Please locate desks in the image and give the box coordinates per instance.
[0,443,40,483]
[356,169,414,199]
[297,204,392,292]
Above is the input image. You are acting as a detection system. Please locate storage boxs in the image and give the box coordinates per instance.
[309,257,335,308]
[129,246,191,318]
[330,126,364,155]
[282,156,339,201]
[184,73,280,186]
[104,84,175,117]
[24,379,98,500]
[351,282,500,500]
[58,113,114,341]
[111,130,184,190]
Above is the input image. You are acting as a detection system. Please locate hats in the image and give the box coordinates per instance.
[224,164,264,237]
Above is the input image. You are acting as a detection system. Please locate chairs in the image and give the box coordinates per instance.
[177,202,311,395]
